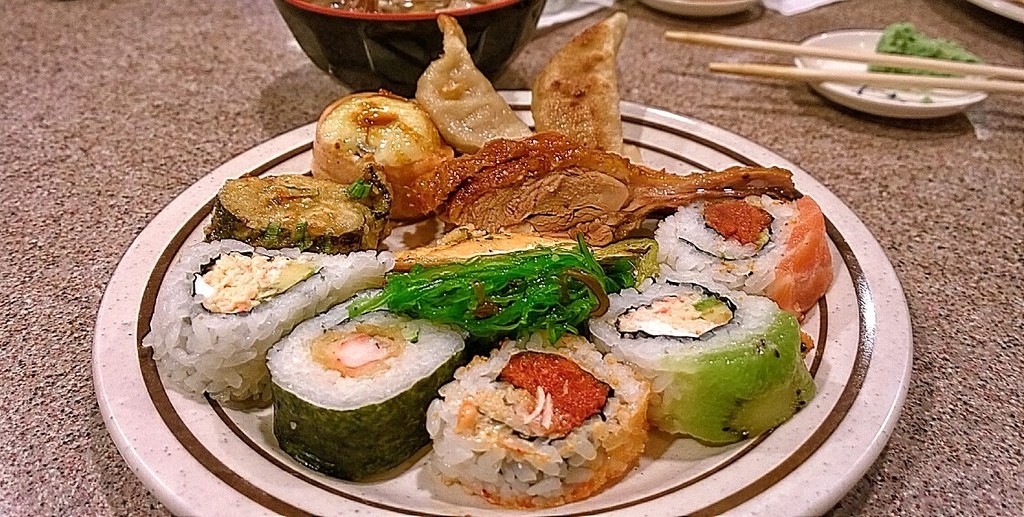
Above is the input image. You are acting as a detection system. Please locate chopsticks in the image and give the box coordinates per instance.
[662,26,1024,107]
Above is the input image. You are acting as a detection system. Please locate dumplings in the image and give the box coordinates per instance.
[416,11,630,156]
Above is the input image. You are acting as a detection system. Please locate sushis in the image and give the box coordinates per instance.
[142,91,835,510]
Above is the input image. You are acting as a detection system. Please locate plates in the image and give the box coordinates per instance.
[91,85,913,517]
[792,29,992,120]
[964,0,1024,22]
[640,0,757,17]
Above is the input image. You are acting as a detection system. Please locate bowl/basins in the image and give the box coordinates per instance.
[272,0,550,102]
